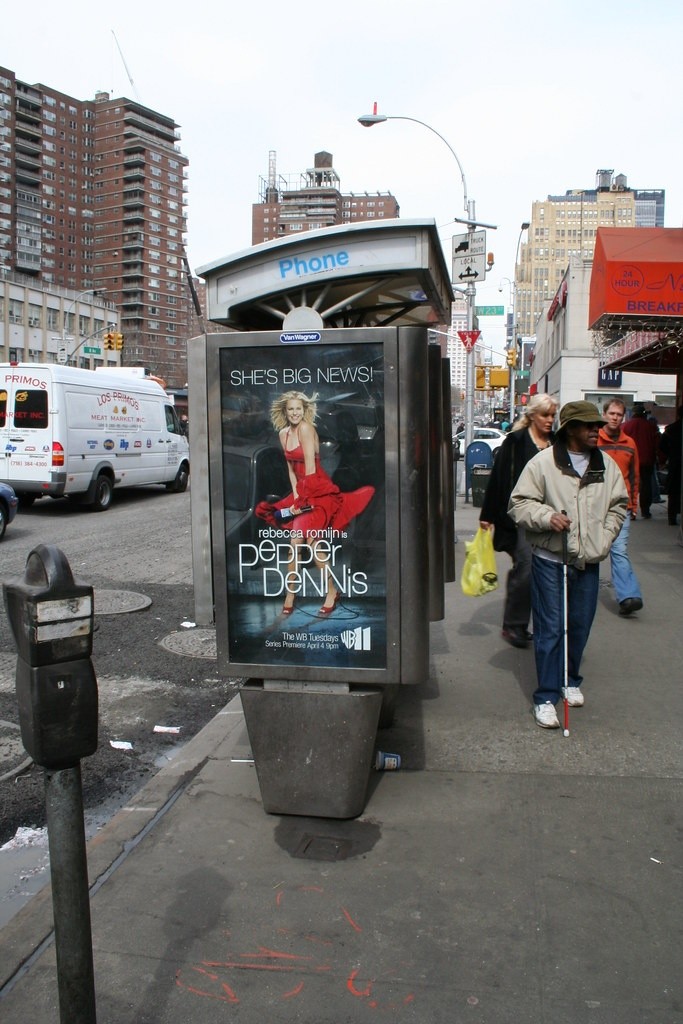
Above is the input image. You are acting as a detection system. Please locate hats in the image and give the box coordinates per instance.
[553,400,609,436]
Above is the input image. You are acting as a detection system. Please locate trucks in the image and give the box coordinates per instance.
[0,361,190,542]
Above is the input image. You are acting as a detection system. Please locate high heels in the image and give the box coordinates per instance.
[282,604,294,614]
[319,592,340,613]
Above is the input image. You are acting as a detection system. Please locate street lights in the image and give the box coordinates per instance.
[357,113,476,503]
[62,287,108,366]
[509,223,529,424]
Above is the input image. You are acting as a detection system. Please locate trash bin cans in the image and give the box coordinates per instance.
[471,468,493,507]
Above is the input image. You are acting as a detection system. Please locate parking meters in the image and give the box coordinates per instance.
[0,543,99,1024]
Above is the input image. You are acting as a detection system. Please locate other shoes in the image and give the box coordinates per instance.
[618,597,643,615]
[501,626,533,647]
[641,511,651,517]
[654,500,666,503]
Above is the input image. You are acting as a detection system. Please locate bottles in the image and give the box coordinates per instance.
[274,505,314,520]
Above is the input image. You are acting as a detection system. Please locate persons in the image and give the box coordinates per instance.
[456,416,516,435]
[479,394,557,648]
[253,392,375,614]
[620,405,683,526]
[598,399,643,616]
[507,400,629,728]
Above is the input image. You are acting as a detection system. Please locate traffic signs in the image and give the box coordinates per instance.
[451,257,485,283]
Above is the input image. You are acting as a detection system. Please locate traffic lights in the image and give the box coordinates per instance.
[103,334,108,350]
[507,349,516,367]
[520,395,530,405]
[108,333,115,350]
[116,333,123,350]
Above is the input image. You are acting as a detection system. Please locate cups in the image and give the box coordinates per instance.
[375,751,401,772]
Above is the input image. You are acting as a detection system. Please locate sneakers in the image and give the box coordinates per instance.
[560,687,584,707]
[530,701,559,728]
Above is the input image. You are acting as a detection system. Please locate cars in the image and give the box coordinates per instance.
[452,428,509,457]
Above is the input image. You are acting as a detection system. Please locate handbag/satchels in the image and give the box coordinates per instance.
[461,526,498,597]
